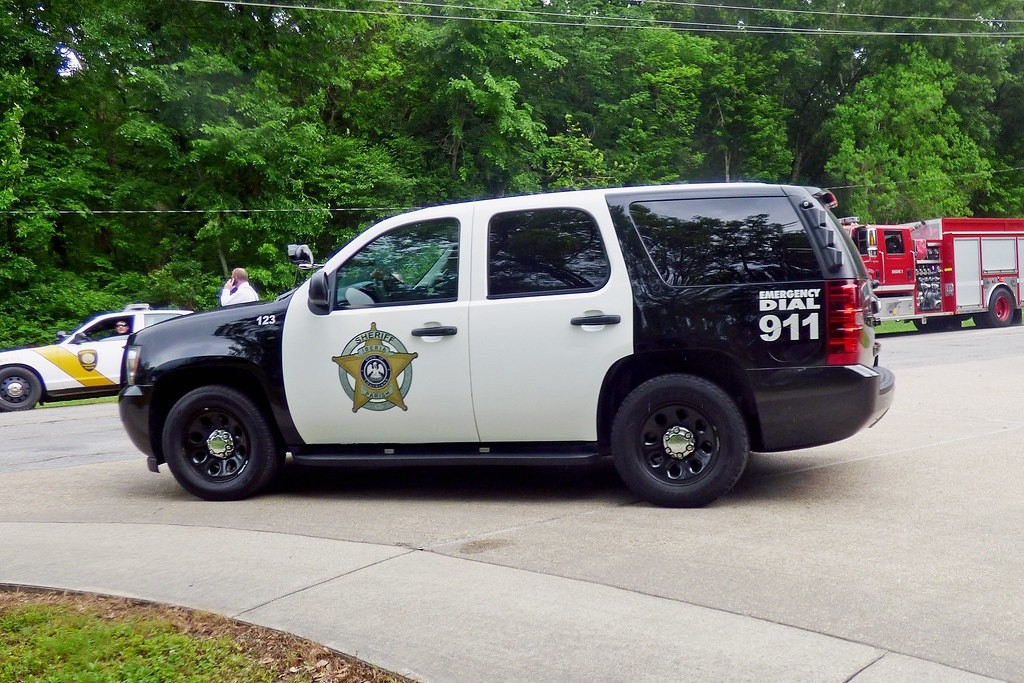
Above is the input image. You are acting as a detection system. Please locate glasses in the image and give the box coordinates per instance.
[116,323,128,327]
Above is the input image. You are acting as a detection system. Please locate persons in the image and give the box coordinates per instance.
[886,236,903,253]
[220,268,259,306]
[371,252,459,302]
[78,318,133,343]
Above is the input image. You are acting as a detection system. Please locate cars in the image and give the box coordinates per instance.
[0,303,195,412]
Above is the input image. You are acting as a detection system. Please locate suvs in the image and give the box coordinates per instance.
[118,181,895,511]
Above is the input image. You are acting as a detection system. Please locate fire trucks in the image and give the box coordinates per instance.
[837,217,1024,333]
